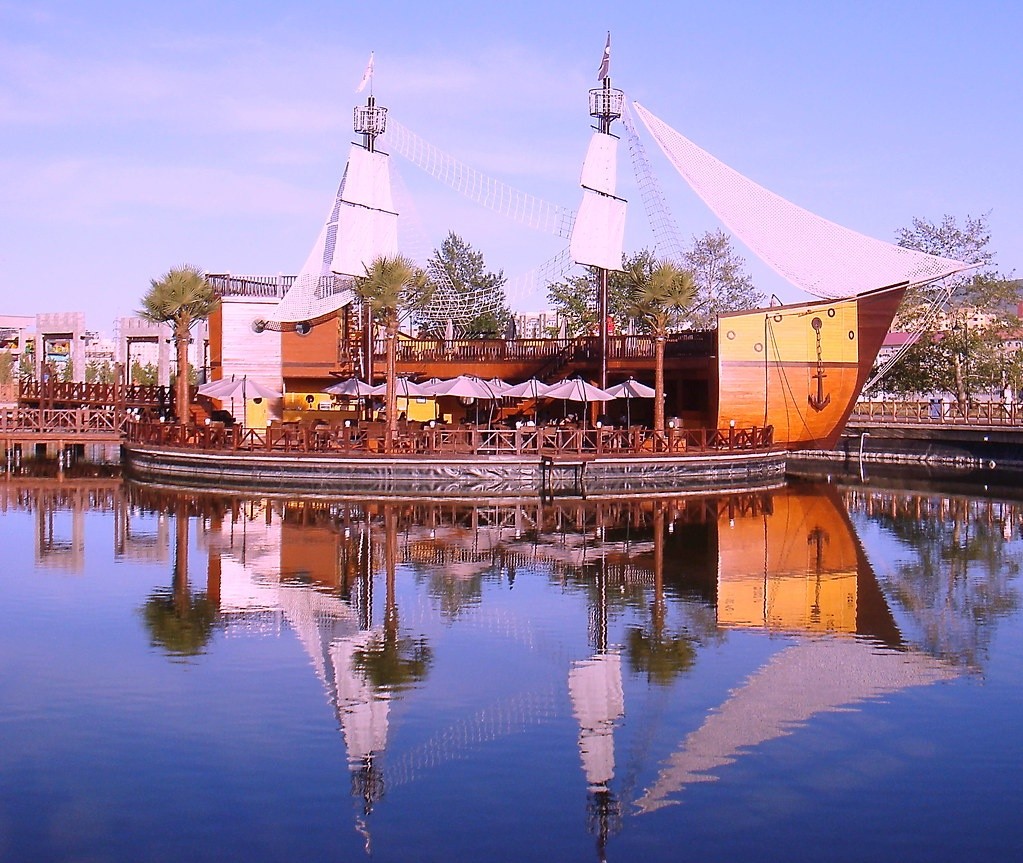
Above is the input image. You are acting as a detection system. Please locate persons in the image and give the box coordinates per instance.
[378,413,386,422]
[515,414,574,446]
[460,417,470,424]
[436,413,448,441]
[373,398,386,412]
[399,412,406,421]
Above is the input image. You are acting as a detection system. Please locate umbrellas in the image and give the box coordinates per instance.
[419,374,512,429]
[445,319,453,360]
[320,378,375,409]
[543,378,617,446]
[537,379,571,418]
[603,379,667,430]
[506,316,515,356]
[197,375,282,445]
[502,379,554,426]
[371,379,437,396]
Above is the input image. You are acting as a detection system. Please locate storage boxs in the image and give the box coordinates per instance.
[656,437,687,452]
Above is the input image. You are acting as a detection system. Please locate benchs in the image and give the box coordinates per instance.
[367,421,418,454]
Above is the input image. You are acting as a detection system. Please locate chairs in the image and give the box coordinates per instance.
[493,423,649,455]
[223,421,340,454]
